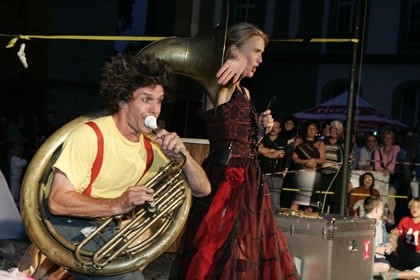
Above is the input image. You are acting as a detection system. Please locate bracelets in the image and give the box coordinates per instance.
[184,150,188,155]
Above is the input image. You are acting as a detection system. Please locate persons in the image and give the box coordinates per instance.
[255,106,420,280]
[0,114,57,202]
[158,119,166,130]
[167,23,300,280]
[43,52,212,279]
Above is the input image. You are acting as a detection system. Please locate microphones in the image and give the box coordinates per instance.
[265,94,277,110]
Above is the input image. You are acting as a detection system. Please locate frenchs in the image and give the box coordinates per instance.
[18,3,231,277]
